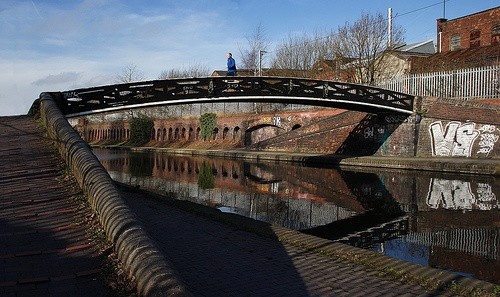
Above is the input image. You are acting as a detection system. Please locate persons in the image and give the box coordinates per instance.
[226,52,237,76]
